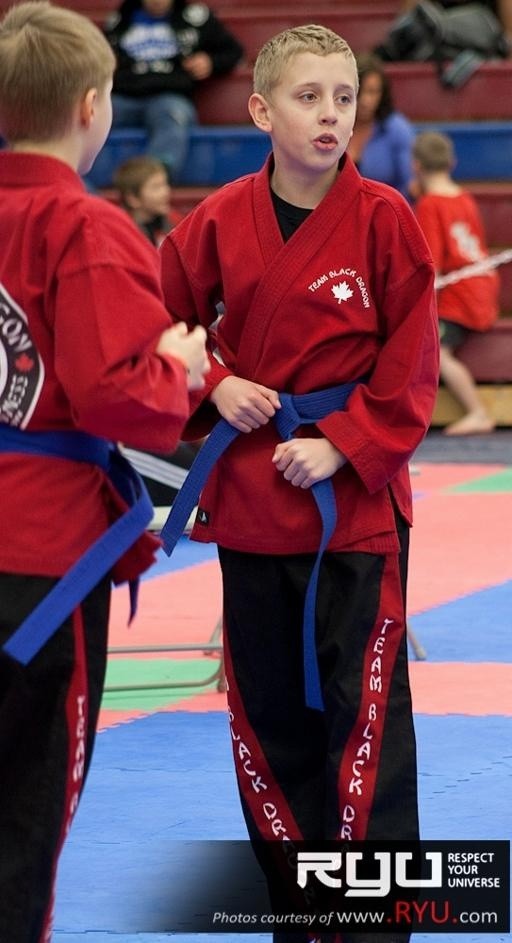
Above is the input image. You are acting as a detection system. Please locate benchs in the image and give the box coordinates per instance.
[0,1,511,431]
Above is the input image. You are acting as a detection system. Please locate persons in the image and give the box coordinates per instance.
[404,132,501,438]
[0,0,438,941]
[355,0,512,62]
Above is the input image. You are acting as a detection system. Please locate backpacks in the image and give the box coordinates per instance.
[371,1,503,91]
